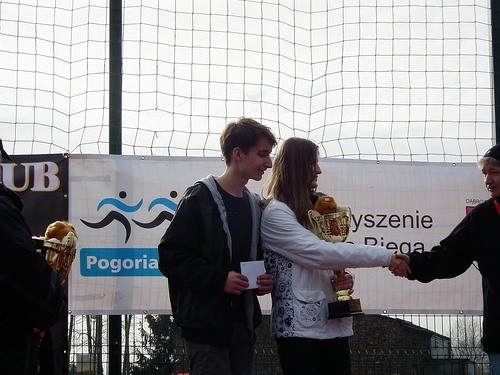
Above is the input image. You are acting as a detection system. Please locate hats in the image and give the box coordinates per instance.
[483,142,500,162]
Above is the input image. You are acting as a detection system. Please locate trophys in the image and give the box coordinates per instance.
[308,195,365,320]
[44,220,77,284]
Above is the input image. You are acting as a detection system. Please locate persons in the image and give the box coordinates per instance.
[159,117,275,375]
[0,182,66,375]
[389,144,500,375]
[262,138,411,375]
